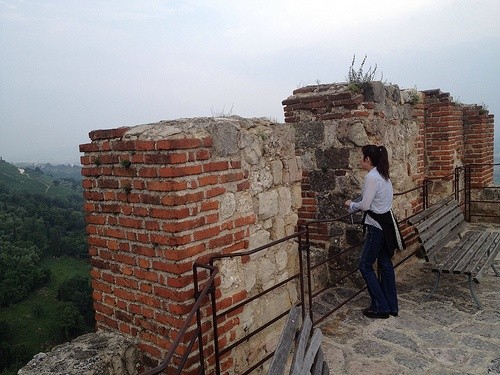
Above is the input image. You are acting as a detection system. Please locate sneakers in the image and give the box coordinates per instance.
[363,306,398,319]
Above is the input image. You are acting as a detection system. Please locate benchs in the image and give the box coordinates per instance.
[408,196,500,310]
[267,305,330,375]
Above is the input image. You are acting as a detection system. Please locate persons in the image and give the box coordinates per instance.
[345,145,405,319]
